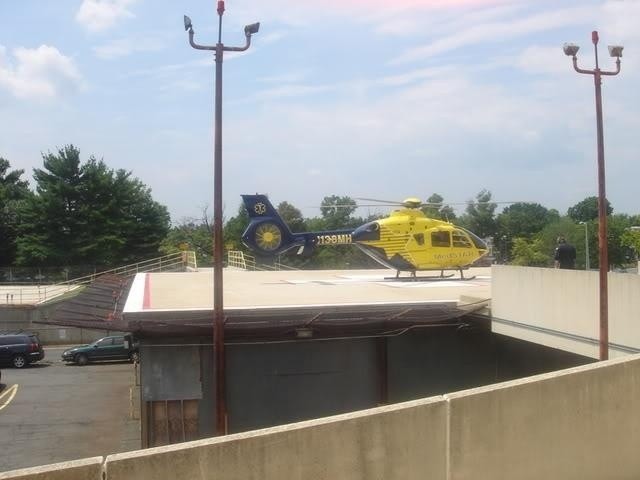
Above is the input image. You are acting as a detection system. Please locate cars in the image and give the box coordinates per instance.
[61,336,140,365]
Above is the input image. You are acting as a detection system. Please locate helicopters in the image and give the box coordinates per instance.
[241,194,523,281]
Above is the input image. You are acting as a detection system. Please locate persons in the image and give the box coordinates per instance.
[554,236,577,269]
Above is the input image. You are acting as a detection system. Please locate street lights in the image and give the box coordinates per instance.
[579,221,590,270]
[564,31,623,360]
[184,1,259,436]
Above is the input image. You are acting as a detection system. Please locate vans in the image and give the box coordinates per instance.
[1,329,45,368]
